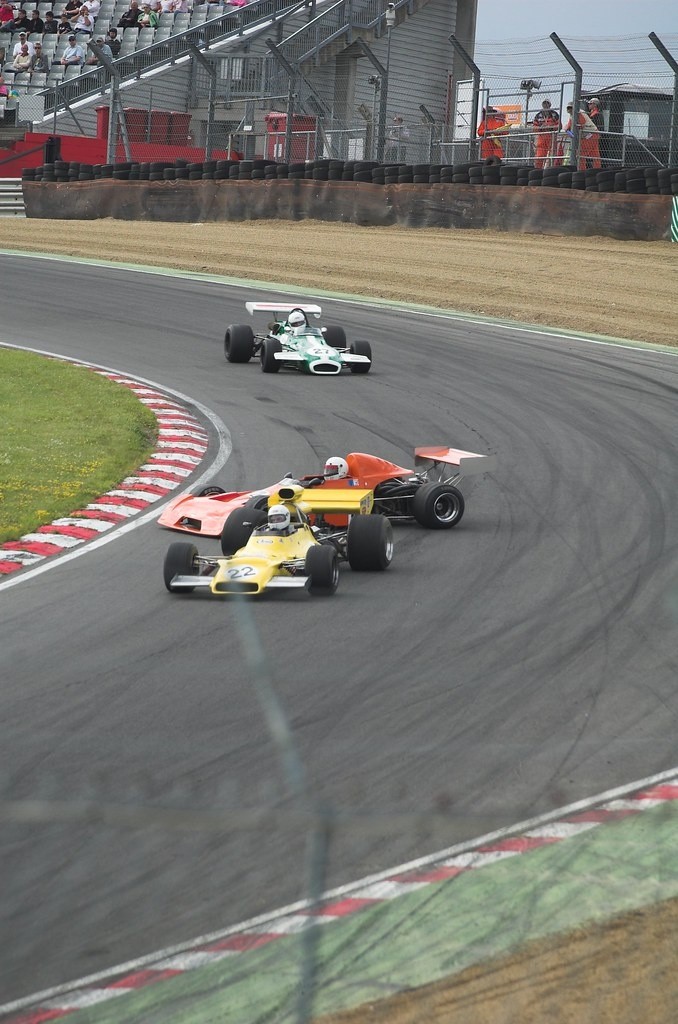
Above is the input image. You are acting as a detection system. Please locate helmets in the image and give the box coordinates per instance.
[323,457,348,481]
[288,312,306,333]
[267,505,290,530]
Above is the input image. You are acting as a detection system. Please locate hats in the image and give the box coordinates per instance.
[19,32,27,35]
[541,99,551,105]
[69,36,76,40]
[587,98,599,104]
[567,102,574,108]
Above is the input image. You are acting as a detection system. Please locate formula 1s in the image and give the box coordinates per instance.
[223,303,373,376]
[156,445,488,539]
[163,484,414,598]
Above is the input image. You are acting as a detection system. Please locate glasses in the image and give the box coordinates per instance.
[588,103,594,104]
[394,120,397,122]
[35,47,41,49]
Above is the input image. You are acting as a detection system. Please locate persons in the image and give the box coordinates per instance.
[0,77,9,97]
[52,36,84,69]
[534,99,562,168]
[288,312,305,335]
[5,32,49,78]
[477,109,511,160]
[565,101,601,170]
[0,0,99,36]
[267,505,290,535]
[117,0,260,31]
[586,99,604,169]
[324,457,348,480]
[86,28,121,78]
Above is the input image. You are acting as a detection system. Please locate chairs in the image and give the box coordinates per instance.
[0,0,258,119]
